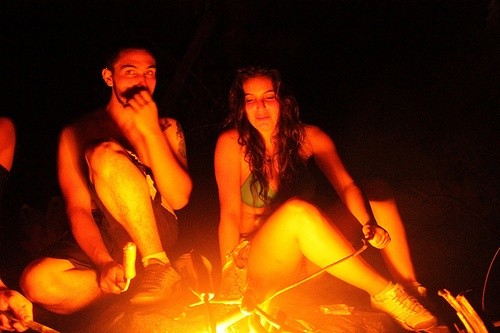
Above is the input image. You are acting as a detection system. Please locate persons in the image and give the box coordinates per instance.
[0,117,33,333]
[214,65,454,333]
[19,47,212,315]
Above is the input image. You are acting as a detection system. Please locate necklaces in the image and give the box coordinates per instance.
[265,154,270,164]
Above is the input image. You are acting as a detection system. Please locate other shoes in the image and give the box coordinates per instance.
[369,282,438,331]
[129,250,213,304]
